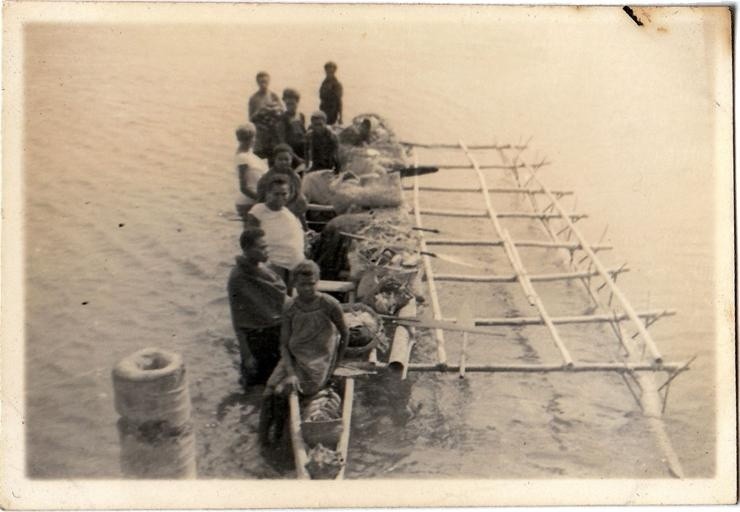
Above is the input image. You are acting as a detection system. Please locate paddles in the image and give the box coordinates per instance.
[340,230,492,269]
[380,316,466,330]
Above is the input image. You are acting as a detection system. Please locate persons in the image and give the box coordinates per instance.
[302,109,342,176]
[233,121,271,226]
[228,227,292,373]
[319,61,345,125]
[245,173,306,296]
[247,70,284,159]
[280,87,307,136]
[257,258,351,440]
[257,141,311,232]
